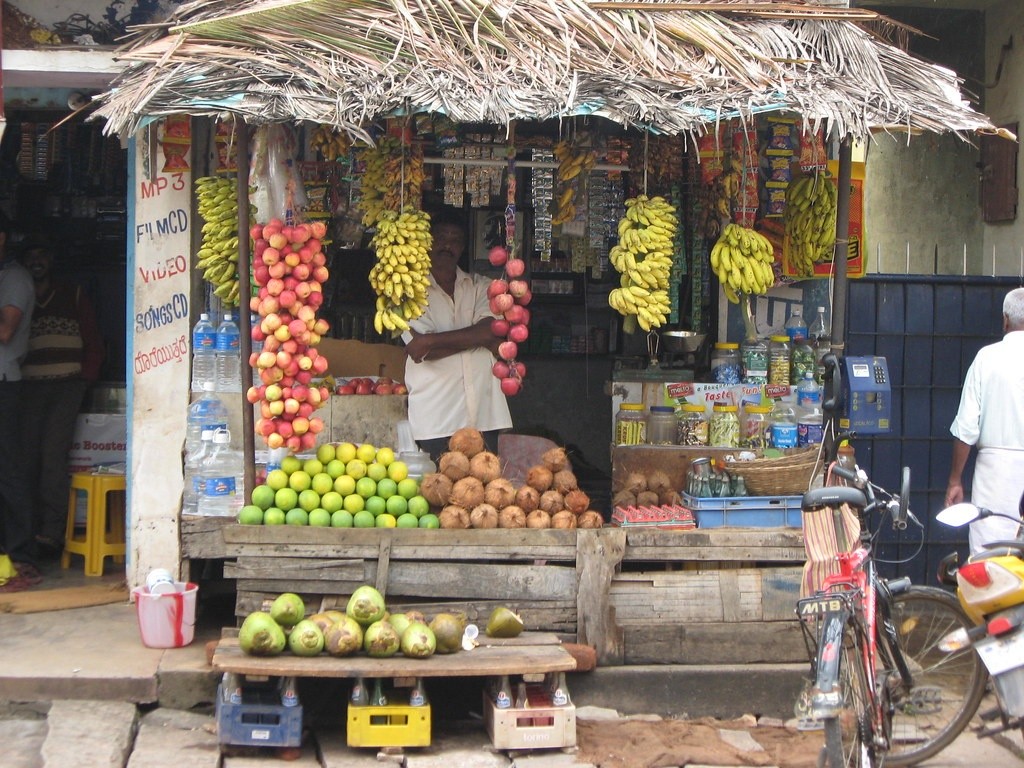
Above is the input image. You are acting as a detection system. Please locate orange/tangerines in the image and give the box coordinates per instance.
[239,443,440,529]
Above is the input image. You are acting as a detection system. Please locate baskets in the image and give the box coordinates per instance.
[724,446,825,496]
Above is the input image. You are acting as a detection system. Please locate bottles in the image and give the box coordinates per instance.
[768,334,792,387]
[783,309,809,342]
[796,402,824,449]
[807,306,830,376]
[647,406,679,446]
[791,338,820,386]
[685,458,750,507]
[740,407,772,449]
[834,447,858,489]
[710,343,742,385]
[796,372,821,412]
[222,669,571,710]
[614,403,647,445]
[677,404,710,447]
[740,337,769,385]
[184,313,294,520]
[708,406,741,447]
[398,450,437,483]
[768,402,797,448]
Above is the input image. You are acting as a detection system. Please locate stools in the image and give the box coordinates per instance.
[60,470,126,577]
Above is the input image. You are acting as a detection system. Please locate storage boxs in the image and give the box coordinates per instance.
[482,684,576,750]
[346,680,432,748]
[680,490,803,528]
[215,682,303,747]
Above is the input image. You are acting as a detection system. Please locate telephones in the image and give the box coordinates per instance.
[821,354,892,436]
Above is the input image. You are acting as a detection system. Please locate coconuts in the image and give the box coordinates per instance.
[237,584,524,658]
[613,472,681,508]
[421,428,603,529]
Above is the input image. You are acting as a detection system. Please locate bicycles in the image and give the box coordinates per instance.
[800,467,989,768]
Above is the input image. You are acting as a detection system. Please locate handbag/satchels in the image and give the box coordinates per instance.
[801,461,862,611]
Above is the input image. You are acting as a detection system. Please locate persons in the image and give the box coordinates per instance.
[401,207,514,473]
[0,224,103,573]
[945,287,1024,558]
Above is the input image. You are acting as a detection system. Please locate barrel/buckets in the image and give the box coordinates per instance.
[130,570,199,647]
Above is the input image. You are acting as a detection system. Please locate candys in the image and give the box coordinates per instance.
[675,412,768,450]
[711,348,816,387]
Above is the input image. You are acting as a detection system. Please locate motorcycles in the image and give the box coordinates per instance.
[934,501,1024,738]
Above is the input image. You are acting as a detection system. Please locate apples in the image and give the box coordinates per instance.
[485,246,533,396]
[337,377,408,395]
[246,218,329,454]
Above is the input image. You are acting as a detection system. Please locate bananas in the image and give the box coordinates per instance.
[784,170,838,278]
[550,138,594,225]
[705,154,776,303]
[361,134,433,334]
[194,176,259,306]
[608,194,680,333]
[309,125,348,160]
